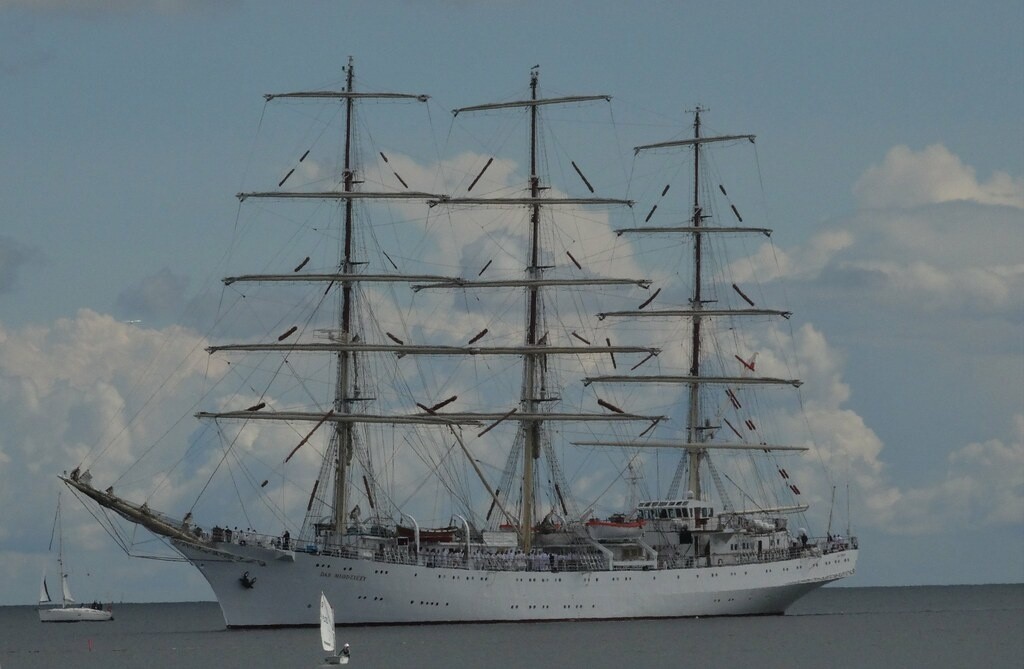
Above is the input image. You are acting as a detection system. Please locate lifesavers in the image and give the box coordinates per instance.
[239,539,247,547]
[452,560,459,569]
[718,558,723,567]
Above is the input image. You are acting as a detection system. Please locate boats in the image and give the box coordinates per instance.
[394,524,457,544]
[498,521,647,544]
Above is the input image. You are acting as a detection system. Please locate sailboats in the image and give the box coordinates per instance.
[320,588,350,666]
[56,53,861,629]
[37,492,112,621]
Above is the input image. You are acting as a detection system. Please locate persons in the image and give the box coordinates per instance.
[420,547,555,571]
[225,526,291,549]
[801,533,842,545]
